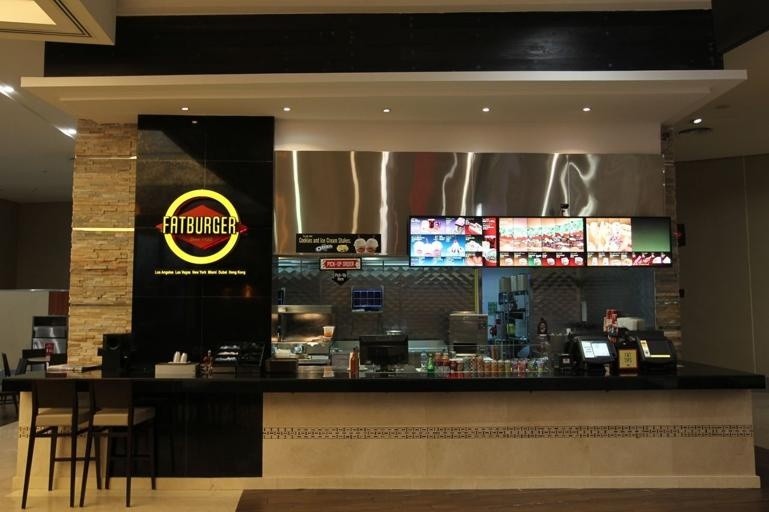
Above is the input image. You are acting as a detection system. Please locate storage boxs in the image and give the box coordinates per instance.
[499,274,528,291]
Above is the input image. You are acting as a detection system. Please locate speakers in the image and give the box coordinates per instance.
[102,332,134,378]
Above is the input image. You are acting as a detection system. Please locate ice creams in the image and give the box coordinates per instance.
[365,239,378,254]
[354,239,366,254]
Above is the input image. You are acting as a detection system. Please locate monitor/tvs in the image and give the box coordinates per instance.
[628,335,678,371]
[359,334,409,373]
[575,335,618,364]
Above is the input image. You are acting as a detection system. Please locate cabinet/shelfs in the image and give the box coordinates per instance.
[489,291,527,345]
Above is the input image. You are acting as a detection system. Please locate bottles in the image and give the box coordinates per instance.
[349,346,360,371]
[426,351,449,372]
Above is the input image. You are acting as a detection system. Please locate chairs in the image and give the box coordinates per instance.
[21,376,157,507]
[0,352,20,417]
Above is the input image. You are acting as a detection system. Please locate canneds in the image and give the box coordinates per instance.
[464,355,526,371]
